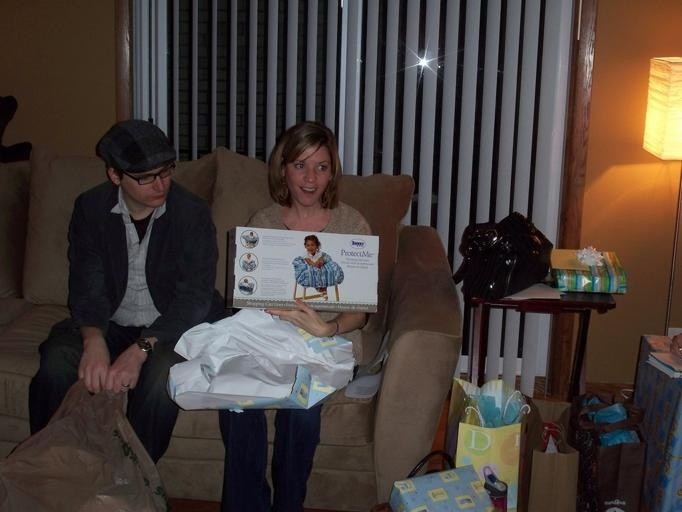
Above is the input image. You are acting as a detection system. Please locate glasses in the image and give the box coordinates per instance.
[119,161,176,186]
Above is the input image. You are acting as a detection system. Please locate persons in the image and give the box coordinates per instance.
[300,235,328,294]
[247,231,254,239]
[26,118,219,465]
[244,253,253,260]
[219,119,374,512]
[243,278,249,283]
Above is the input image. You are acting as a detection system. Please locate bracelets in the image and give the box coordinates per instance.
[327,319,339,338]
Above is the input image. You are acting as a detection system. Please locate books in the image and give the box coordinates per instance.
[646,351,682,379]
[550,248,591,272]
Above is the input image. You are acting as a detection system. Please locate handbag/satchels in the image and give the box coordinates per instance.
[459,212,553,303]
[387,377,647,511]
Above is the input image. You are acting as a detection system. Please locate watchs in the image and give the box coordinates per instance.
[135,337,152,354]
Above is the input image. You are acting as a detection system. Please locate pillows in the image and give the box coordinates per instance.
[23,141,217,308]
[209,146,414,361]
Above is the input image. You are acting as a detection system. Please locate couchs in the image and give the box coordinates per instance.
[0,145,464,511]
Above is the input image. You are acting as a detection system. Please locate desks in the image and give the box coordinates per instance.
[625,333,681,511]
[468,279,616,409]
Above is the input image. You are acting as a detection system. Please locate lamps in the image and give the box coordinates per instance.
[643,55,682,339]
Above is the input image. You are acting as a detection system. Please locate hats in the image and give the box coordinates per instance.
[98,119,177,174]
